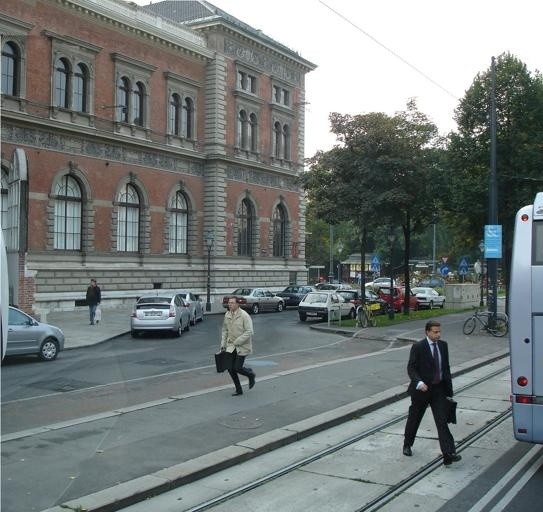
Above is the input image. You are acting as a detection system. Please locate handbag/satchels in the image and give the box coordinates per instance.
[215,350,226,372]
[447,398,457,424]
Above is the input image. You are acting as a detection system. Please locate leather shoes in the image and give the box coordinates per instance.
[249,374,254,388]
[444,455,461,464]
[403,446,412,456]
[232,392,242,396]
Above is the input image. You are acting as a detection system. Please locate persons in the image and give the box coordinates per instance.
[221,296,255,396]
[86,280,101,325]
[356,272,361,286]
[403,321,461,465]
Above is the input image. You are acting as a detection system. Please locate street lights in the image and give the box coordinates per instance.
[479,240,485,306]
[203,235,213,312]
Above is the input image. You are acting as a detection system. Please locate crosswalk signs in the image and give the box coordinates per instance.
[458,257,469,275]
[371,257,380,272]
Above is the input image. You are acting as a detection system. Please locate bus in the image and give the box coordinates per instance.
[508,192,543,443]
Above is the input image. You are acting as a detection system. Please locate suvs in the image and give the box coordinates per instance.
[364,277,396,289]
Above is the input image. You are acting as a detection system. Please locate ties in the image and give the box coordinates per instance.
[432,343,440,385]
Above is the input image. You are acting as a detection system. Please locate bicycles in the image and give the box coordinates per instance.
[355,302,377,328]
[463,305,508,337]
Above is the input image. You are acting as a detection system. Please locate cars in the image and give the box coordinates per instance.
[278,284,313,306]
[130,293,204,337]
[299,291,356,321]
[315,283,445,314]
[5,306,64,361]
[222,288,285,312]
